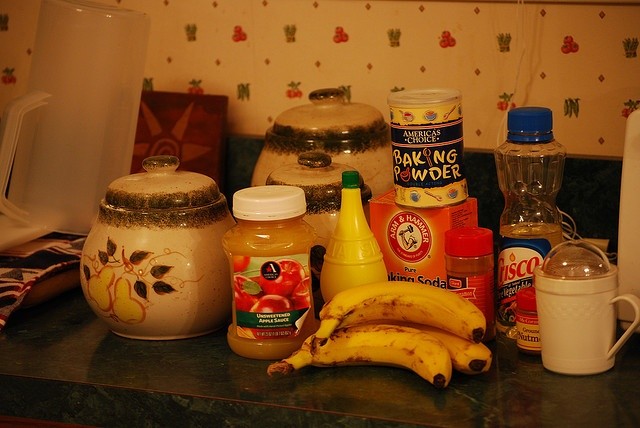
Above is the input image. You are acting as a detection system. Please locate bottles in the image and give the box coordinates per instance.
[494,106,567,333]
[515,287,540,358]
[320,170,388,302]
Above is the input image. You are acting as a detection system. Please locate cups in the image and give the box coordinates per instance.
[534,240,640,375]
[0,0,152,237]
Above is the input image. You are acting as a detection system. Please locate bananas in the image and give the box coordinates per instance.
[310,280,487,360]
[405,323,492,376]
[268,325,452,390]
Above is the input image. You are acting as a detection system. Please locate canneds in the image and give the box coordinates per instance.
[516,285,544,363]
[388,89,469,209]
